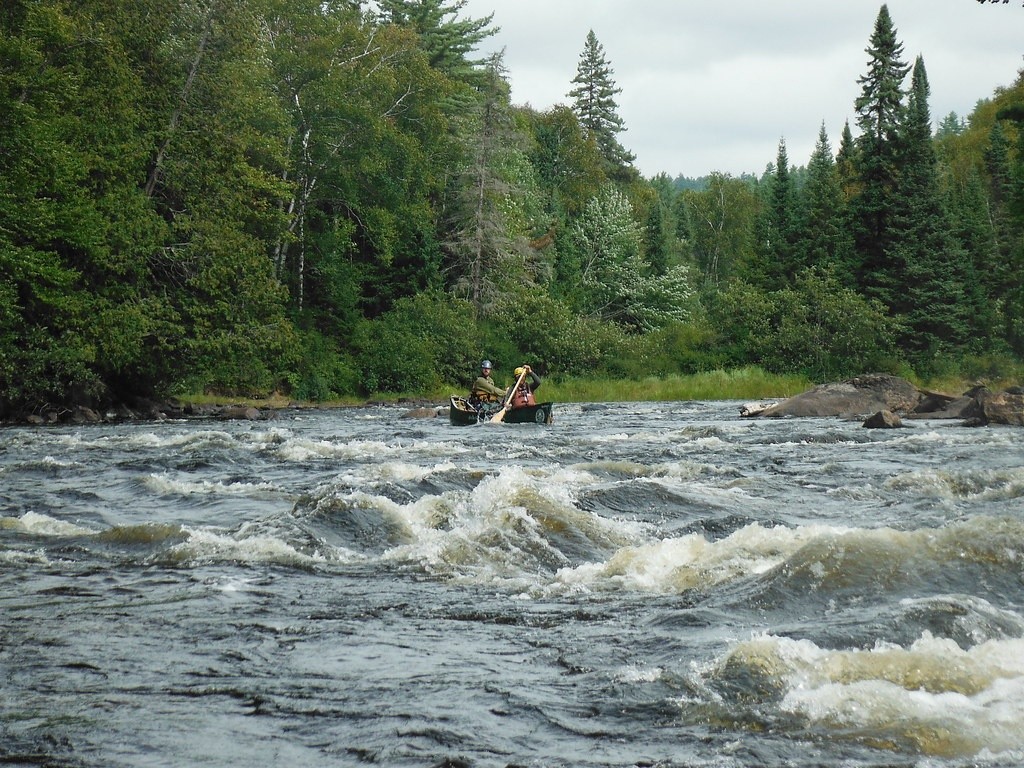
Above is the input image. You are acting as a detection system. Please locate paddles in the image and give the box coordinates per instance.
[489,367,528,423]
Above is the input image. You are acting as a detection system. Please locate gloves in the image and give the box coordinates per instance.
[522,365,532,375]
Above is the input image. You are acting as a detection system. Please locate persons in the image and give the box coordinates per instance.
[504,365,542,411]
[469,360,509,410]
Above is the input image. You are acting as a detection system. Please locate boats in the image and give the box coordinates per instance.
[450,392,555,427]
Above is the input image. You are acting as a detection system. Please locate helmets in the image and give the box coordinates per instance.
[513,367,526,377]
[481,360,492,368]
